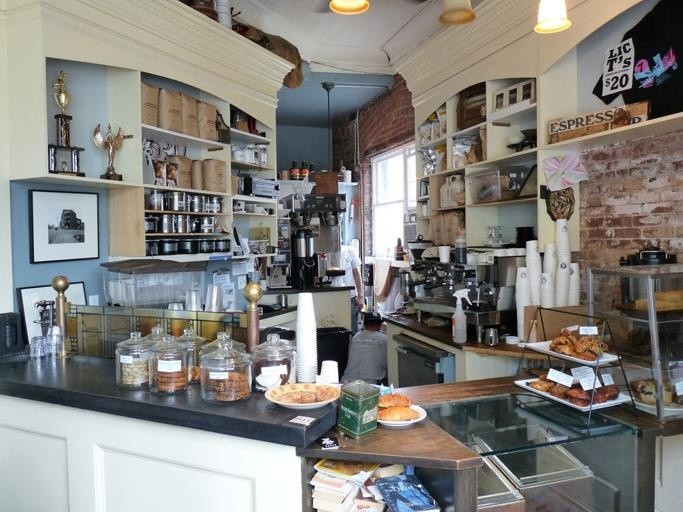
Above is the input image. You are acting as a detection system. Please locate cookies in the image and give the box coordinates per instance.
[251,360,284,394]
[213,372,249,402]
[155,369,187,392]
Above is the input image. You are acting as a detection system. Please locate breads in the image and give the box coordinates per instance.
[628,376,675,406]
[530,371,618,408]
[376,395,417,422]
[553,331,610,361]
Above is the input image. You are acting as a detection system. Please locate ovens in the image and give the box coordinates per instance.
[394,346,442,388]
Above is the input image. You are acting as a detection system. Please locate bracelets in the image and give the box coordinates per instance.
[358,293,363,298]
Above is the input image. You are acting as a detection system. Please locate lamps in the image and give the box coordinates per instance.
[436,0,476,26]
[528,0,572,33]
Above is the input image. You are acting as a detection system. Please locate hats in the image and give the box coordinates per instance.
[362,311,384,324]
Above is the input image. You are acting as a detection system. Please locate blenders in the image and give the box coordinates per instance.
[408,239,432,270]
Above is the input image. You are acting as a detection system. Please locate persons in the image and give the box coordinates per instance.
[339,311,389,386]
[331,244,363,336]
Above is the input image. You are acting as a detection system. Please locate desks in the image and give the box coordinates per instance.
[416,379,640,512]
[296,421,483,512]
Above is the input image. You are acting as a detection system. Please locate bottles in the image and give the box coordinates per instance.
[455,236,465,263]
[290,160,315,180]
[111,327,294,405]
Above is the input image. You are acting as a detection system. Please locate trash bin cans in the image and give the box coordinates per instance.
[317,327,350,383]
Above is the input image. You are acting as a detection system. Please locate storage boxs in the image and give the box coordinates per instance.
[462,166,527,205]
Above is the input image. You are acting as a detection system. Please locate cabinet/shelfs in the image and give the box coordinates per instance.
[277,175,317,263]
[9,121,231,263]
[586,264,681,425]
[16,281,88,350]
[230,128,278,259]
[416,72,543,264]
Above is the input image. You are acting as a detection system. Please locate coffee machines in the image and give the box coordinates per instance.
[290,230,319,285]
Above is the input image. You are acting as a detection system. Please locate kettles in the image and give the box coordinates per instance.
[620,251,676,341]
[0,311,22,355]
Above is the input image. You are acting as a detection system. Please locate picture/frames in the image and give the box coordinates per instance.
[419,181,428,197]
[511,164,536,200]
[26,188,101,264]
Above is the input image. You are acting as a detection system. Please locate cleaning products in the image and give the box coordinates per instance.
[449,288,473,344]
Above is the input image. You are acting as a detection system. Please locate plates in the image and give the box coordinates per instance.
[267,382,343,411]
[381,403,428,429]
[511,227,536,247]
[513,376,632,410]
[526,338,622,366]
[627,394,683,413]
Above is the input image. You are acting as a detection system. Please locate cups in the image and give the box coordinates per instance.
[279,169,289,180]
[486,328,498,345]
[25,327,65,364]
[295,292,337,386]
[170,284,224,311]
[439,245,450,264]
[342,170,350,183]
[258,279,268,289]
[517,221,581,340]
